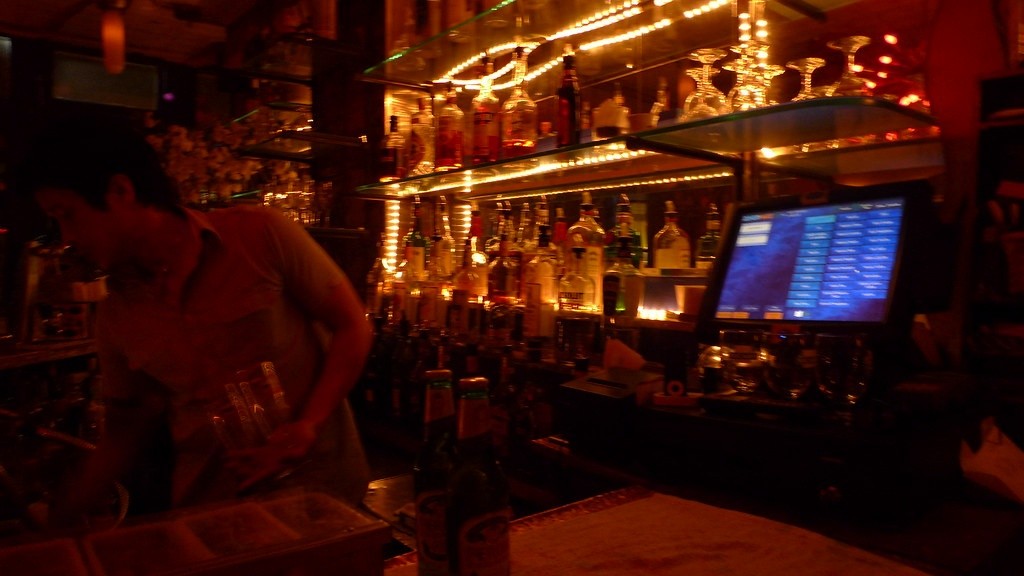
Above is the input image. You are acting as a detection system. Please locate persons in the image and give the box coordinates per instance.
[15,102,376,539]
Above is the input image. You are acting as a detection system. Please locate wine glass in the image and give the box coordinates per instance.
[810,85,843,149]
[826,34,876,147]
[684,48,785,123]
[785,57,827,154]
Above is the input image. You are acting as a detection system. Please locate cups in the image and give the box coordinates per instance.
[702,329,870,404]
[674,285,707,315]
[603,271,641,319]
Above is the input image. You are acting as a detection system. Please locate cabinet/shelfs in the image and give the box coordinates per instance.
[347,1,942,206]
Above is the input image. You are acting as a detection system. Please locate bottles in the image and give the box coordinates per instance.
[436,95,465,172]
[399,191,604,320]
[552,55,583,149]
[412,369,456,576]
[407,98,436,178]
[379,116,405,183]
[365,312,639,426]
[446,376,508,576]
[696,204,721,267]
[500,47,538,167]
[604,195,642,268]
[650,77,670,127]
[466,58,500,166]
[653,201,690,269]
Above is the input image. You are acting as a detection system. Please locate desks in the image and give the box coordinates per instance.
[383,486,931,576]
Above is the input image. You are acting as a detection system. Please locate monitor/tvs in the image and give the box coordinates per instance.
[696,180,934,347]
